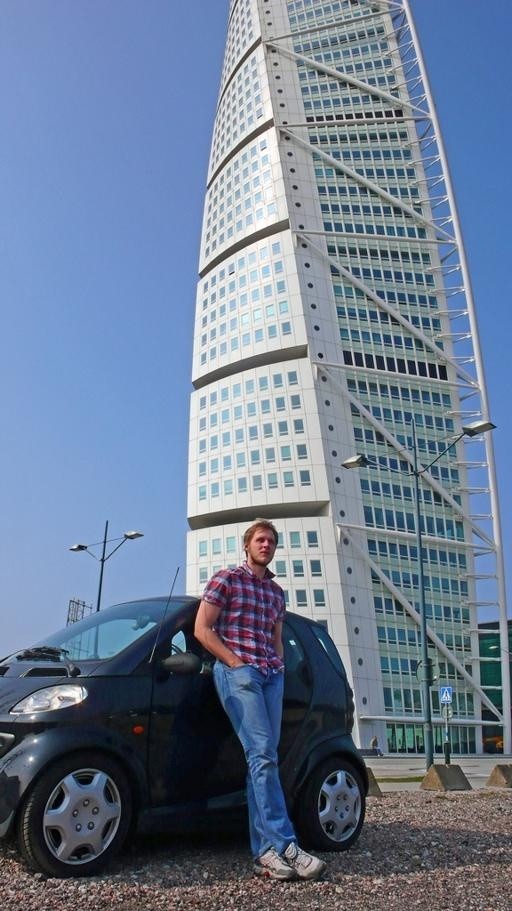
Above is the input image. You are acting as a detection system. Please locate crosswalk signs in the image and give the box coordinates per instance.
[439,685,453,703]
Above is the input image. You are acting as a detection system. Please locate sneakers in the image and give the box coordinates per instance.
[251,845,296,882]
[281,841,329,881]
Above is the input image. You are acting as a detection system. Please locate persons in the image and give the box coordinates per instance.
[370,736,384,758]
[191,517,329,886]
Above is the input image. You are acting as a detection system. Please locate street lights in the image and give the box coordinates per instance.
[67,519,143,660]
[342,418,497,786]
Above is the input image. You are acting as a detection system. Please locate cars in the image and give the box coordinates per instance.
[1,590,373,886]
